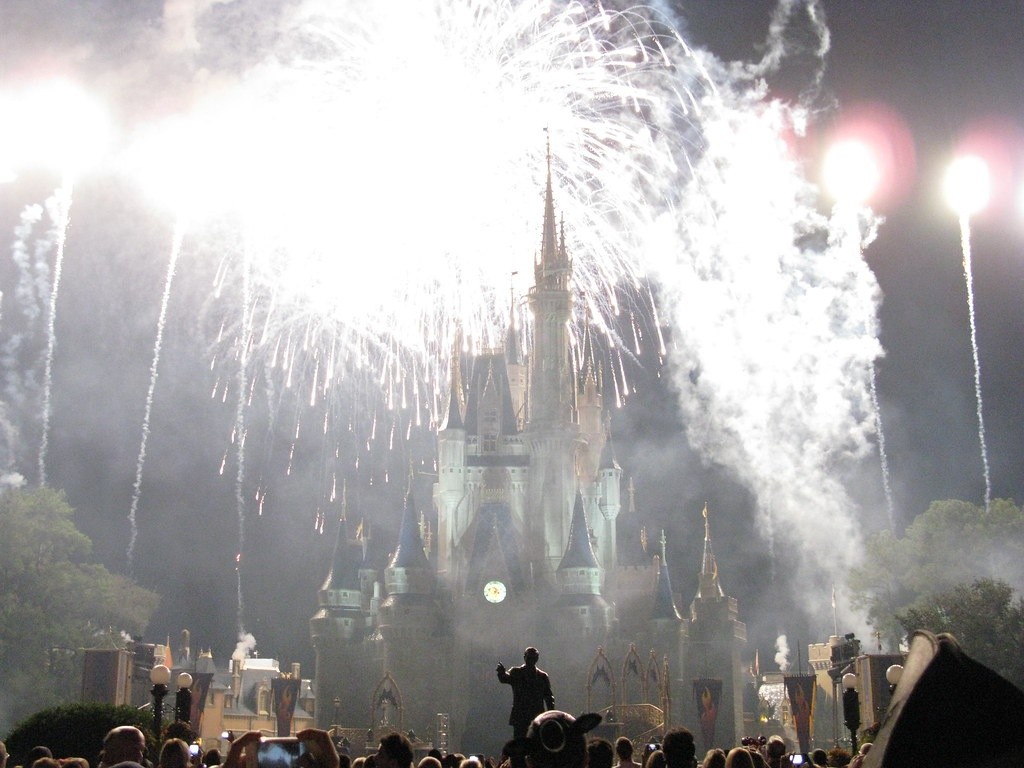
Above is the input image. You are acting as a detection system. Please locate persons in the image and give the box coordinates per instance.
[0,710,876,768]
[495,646,556,740]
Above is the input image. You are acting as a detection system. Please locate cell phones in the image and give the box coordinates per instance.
[469,754,479,761]
[789,755,805,765]
[222,731,229,739]
[189,744,199,759]
[245,736,308,768]
[646,744,659,754]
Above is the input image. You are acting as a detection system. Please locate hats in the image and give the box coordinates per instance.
[502,710,602,768]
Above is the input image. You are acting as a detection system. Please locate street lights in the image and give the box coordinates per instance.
[841,672,861,755]
[149,665,173,743]
[175,672,193,731]
